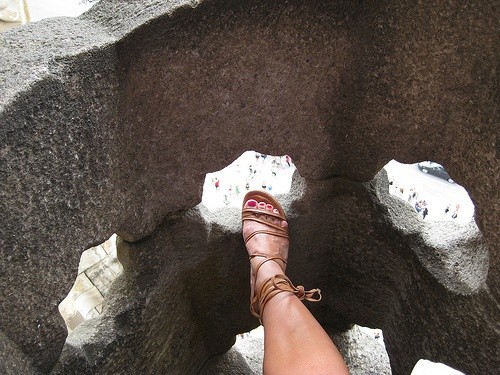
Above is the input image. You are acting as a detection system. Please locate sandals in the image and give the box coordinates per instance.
[241,189,323,327]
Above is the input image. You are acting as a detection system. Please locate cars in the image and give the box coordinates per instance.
[418,162,456,184]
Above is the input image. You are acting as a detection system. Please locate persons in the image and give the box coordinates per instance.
[241,190,352,375]
[210,151,475,225]
